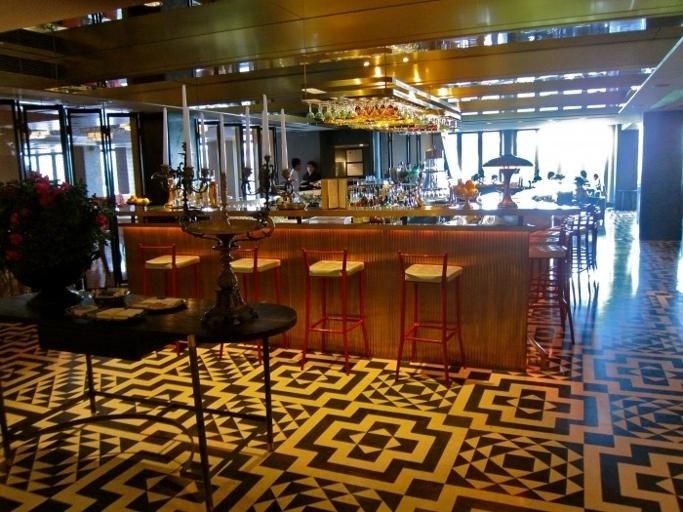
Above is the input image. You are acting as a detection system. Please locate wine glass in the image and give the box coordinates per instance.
[303,96,458,137]
[455,191,482,210]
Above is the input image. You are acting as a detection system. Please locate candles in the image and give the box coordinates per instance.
[159,82,292,181]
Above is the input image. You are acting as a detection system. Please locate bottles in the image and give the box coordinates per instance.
[209,168,218,206]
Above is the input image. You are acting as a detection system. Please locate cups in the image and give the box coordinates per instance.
[351,161,422,208]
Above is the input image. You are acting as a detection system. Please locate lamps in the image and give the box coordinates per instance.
[483,154,532,210]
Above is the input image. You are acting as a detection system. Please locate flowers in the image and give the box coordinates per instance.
[0,168,129,268]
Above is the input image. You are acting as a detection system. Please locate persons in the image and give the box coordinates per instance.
[288,157,300,191]
[593,173,600,186]
[579,169,589,182]
[301,162,322,188]
[546,171,554,180]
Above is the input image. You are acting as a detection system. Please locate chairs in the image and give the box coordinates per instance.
[528,173,607,364]
[297,244,376,381]
[137,242,201,358]
[387,248,468,390]
[217,242,284,367]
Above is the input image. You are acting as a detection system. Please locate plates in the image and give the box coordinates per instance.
[276,202,307,210]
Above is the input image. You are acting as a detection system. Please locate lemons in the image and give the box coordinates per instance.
[453,180,478,194]
[129,195,150,205]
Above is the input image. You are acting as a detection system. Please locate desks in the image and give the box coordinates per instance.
[1,285,302,512]
[615,188,638,210]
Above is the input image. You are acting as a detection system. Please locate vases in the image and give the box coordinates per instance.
[12,267,90,314]
